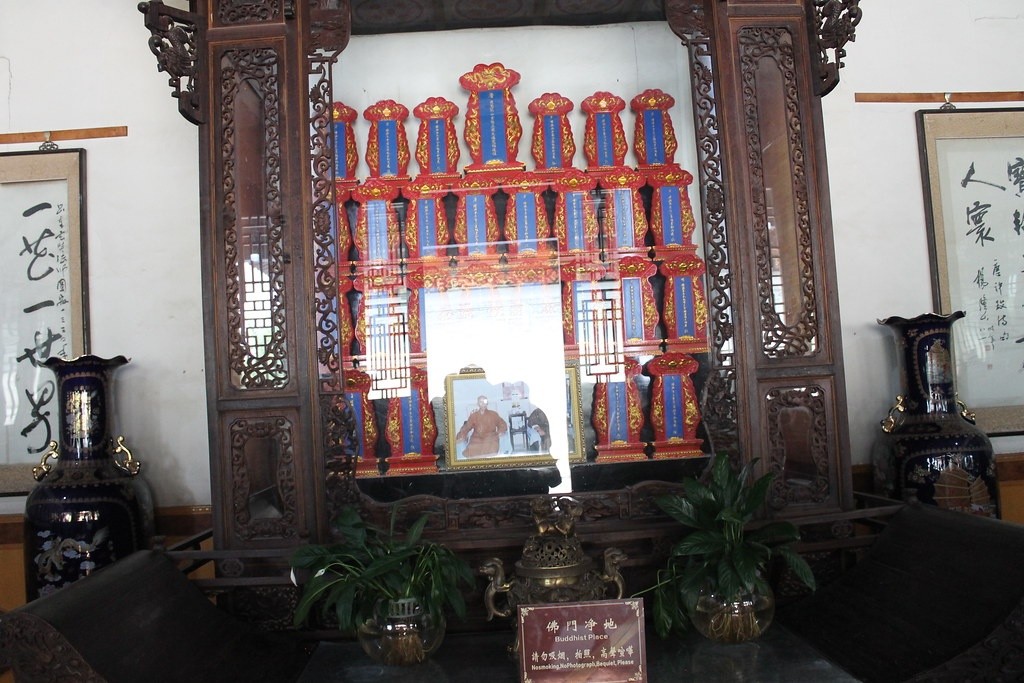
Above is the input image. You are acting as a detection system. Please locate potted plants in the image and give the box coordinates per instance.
[289,501,477,666]
[628,450,819,646]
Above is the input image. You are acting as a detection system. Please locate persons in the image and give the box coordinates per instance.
[457,396,508,458]
[527,407,575,453]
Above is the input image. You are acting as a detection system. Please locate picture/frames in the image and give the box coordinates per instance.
[444,366,588,471]
[0,147,88,498]
[915,107,1024,437]
